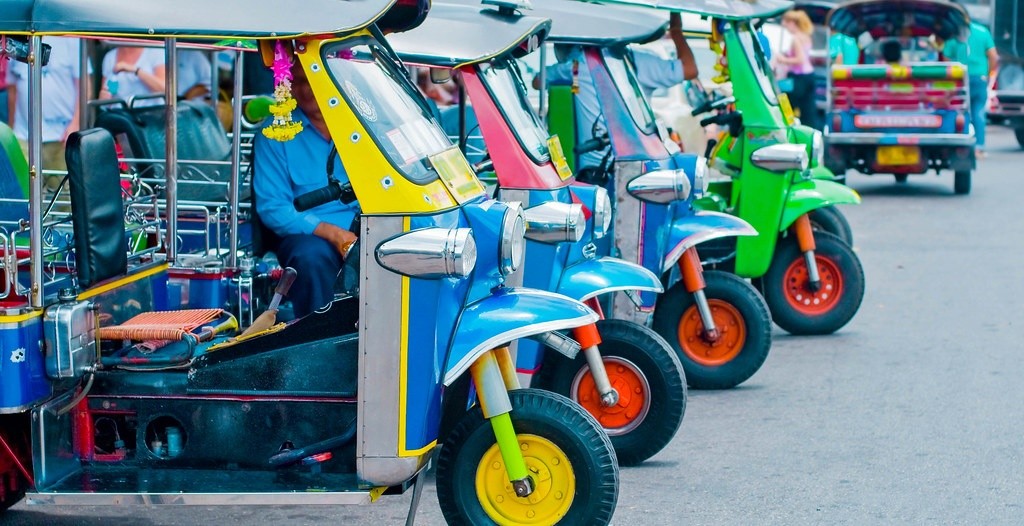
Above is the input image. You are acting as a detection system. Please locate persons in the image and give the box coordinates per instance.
[776,10,816,126]
[97,45,166,108]
[881,42,901,62]
[532,13,698,186]
[251,53,361,319]
[943,12,999,158]
[438,104,487,165]
[176,49,211,101]
[0,34,91,222]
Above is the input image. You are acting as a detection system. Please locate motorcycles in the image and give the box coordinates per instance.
[0,1,620,526]
[823,1,977,195]
[1,2,865,467]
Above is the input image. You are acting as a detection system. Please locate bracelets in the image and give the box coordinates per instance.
[135,68,141,76]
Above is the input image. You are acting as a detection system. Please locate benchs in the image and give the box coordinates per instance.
[89,92,253,203]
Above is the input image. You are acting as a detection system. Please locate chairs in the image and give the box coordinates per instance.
[66,114,242,372]
